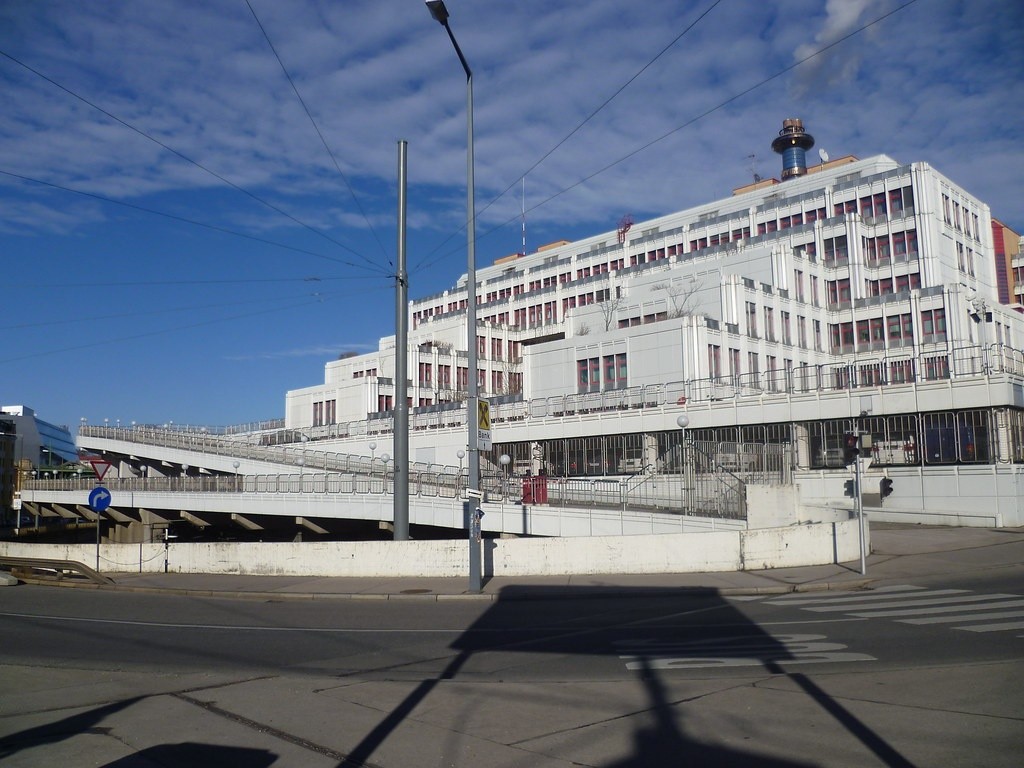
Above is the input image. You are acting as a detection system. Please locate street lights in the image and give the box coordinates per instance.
[424,0,482,593]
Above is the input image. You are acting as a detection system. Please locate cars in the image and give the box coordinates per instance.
[510,417,981,476]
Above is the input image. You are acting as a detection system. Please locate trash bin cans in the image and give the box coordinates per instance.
[523,476,548,503]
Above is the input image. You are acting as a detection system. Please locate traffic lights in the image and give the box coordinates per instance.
[842,433,859,464]
[843,479,857,498]
[880,476,894,499]
